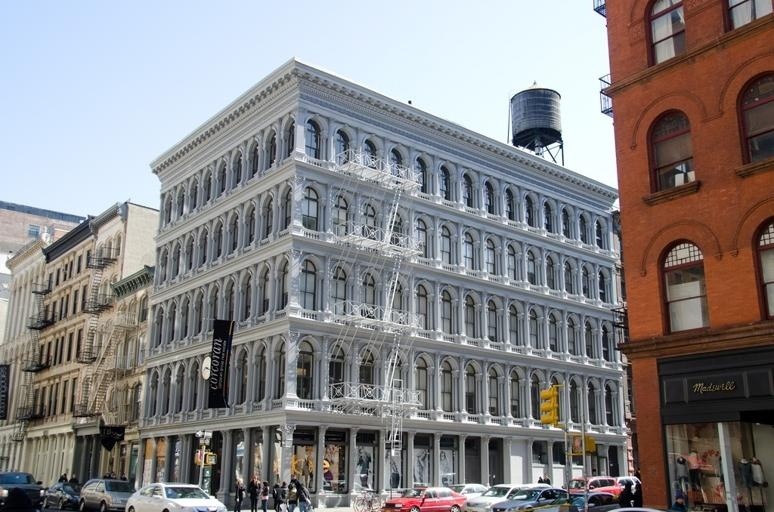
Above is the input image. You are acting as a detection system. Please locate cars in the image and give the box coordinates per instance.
[452,483,487,494]
[532,493,620,512]
[0,472,46,507]
[490,487,570,512]
[383,487,467,512]
[123,481,228,512]
[607,507,676,512]
[43,482,79,511]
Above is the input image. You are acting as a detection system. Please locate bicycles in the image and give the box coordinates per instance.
[354,489,381,512]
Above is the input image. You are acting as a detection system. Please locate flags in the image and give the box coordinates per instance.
[0,364,11,421]
[206,318,235,409]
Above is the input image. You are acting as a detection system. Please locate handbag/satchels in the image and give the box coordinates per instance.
[306,498,311,505]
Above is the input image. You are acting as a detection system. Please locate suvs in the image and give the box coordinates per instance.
[78,479,134,512]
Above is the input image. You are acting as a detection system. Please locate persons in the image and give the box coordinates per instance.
[538,476,544,483]
[618,482,634,508]
[672,498,688,512]
[672,481,688,505]
[633,484,643,508]
[301,446,451,493]
[233,474,310,512]
[544,475,551,485]
[58,470,128,484]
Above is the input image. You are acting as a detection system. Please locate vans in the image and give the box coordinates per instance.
[615,476,642,494]
[562,475,624,502]
[463,484,552,512]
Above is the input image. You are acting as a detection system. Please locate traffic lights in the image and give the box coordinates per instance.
[540,388,556,425]
[571,434,596,455]
[205,453,217,466]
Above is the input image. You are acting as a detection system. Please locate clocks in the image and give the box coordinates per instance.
[202,355,211,381]
